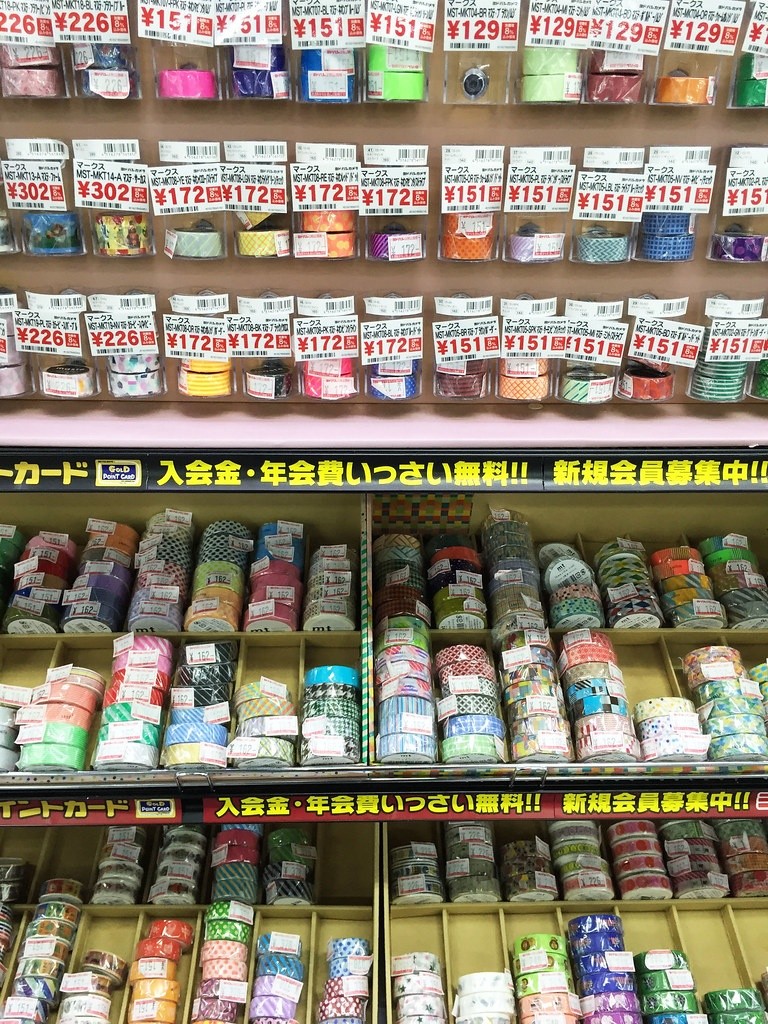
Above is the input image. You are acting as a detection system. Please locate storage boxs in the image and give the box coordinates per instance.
[0,817,768,1024]
[0,490,768,785]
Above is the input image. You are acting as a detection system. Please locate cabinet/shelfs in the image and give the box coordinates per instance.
[0,403,768,1024]
